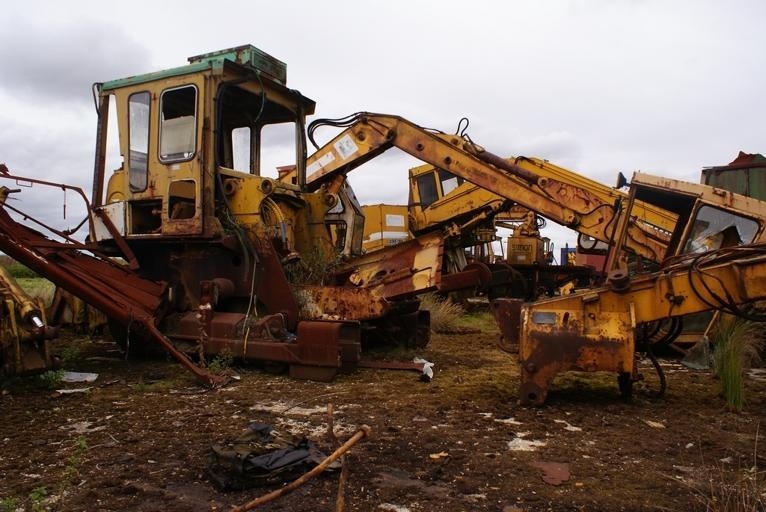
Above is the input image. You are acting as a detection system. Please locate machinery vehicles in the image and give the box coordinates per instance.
[275,111,766,407]
[0,42,446,387]
[360,162,736,353]
